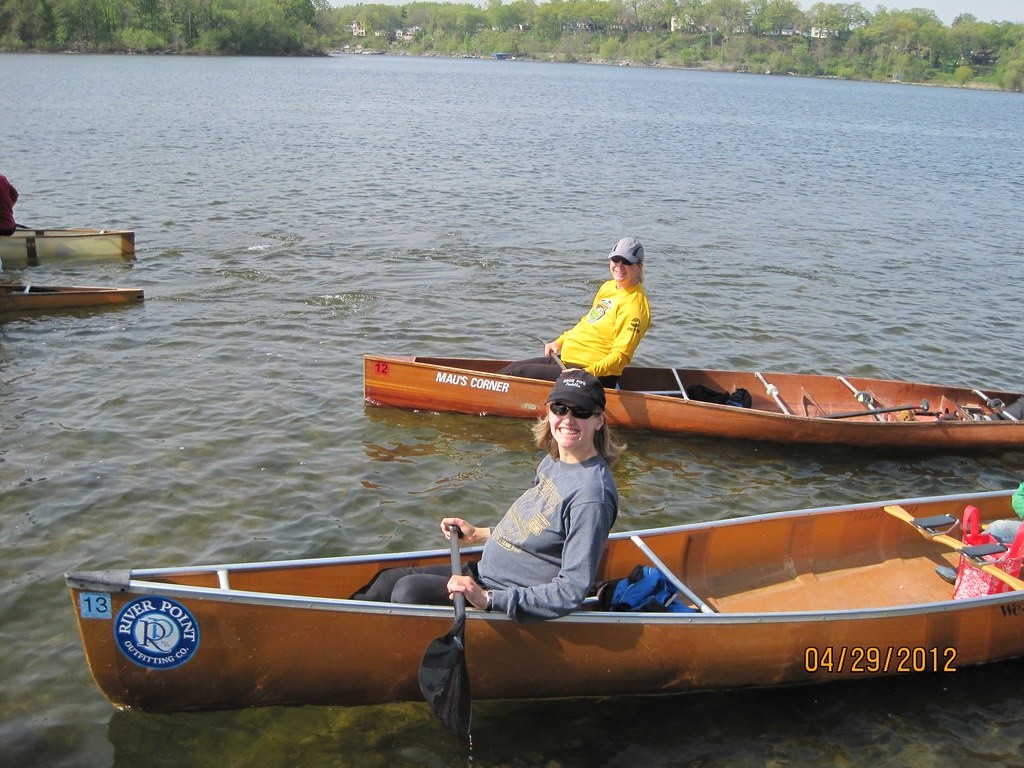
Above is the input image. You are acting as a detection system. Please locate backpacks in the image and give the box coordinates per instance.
[594,564,695,613]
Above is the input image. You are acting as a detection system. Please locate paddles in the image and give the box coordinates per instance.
[516,331,571,375]
[419,526,474,742]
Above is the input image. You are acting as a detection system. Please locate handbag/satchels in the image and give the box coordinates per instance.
[951,506,1024,599]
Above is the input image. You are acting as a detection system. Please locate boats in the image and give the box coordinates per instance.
[62,486,1024,712]
[0,283,145,313]
[364,352,1024,452]
[0,225,136,268]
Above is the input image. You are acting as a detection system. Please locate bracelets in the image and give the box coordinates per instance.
[485,591,493,612]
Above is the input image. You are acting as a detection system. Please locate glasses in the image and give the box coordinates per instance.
[547,402,602,419]
[611,257,640,265]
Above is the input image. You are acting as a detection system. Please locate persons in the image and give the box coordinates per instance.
[493,236,651,388]
[935,482,1024,586]
[350,370,627,624]
[0,174,18,236]
[972,395,1024,421]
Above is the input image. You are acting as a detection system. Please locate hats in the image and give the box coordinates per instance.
[544,369,606,414]
[608,237,644,263]
